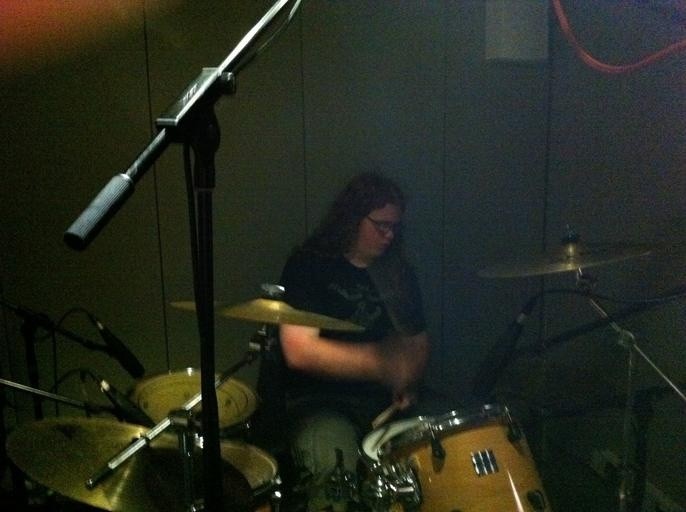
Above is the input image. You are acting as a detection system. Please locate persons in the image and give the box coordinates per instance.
[250,173,430,512]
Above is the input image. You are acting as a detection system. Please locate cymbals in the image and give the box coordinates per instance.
[4,416,254,508]
[170,296,365,332]
[476,239,652,282]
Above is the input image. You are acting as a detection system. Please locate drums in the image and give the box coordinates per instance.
[128,366,261,438]
[193,436,281,508]
[360,415,439,507]
[378,405,552,507]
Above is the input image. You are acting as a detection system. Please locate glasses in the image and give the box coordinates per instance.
[367,216,401,232]
